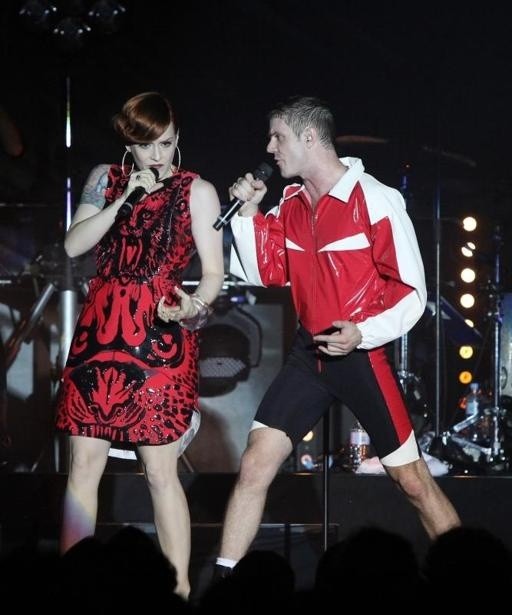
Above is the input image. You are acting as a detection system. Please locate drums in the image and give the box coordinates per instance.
[397,369,432,438]
[349,429,372,471]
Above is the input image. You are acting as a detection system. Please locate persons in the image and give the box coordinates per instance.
[1,522,511,614]
[209,93,463,581]
[55,91,224,602]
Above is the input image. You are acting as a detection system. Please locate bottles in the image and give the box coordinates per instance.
[350,417,370,469]
[464,382,481,416]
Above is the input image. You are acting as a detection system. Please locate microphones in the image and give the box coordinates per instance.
[115,167,159,222]
[213,161,274,230]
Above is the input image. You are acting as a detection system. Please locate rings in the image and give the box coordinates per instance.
[136,175,140,180]
[239,178,244,185]
[234,182,238,188]
